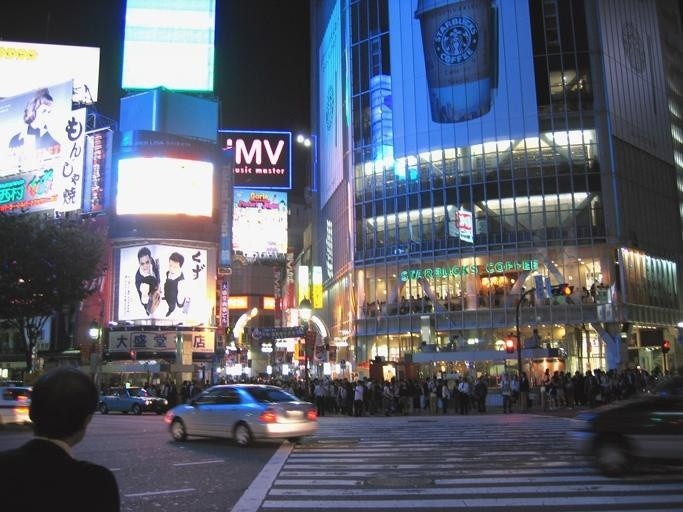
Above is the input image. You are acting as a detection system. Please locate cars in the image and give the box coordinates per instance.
[163,383,318,447]
[0,386,33,428]
[96,385,167,415]
[565,373,682,478]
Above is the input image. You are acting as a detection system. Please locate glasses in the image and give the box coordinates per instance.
[140,260,149,265]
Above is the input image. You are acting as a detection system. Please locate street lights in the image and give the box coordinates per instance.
[297,296,313,401]
[23,323,38,374]
[87,319,100,386]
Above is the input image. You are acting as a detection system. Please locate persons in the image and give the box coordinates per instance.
[0,364,120,512]
[362,280,609,318]
[9,86,60,151]
[135,246,162,318]
[98,365,682,418]
[162,252,187,316]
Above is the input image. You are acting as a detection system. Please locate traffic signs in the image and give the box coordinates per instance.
[545,278,553,298]
[534,275,544,300]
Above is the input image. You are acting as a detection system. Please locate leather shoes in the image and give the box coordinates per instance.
[162,297,166,300]
[166,311,171,316]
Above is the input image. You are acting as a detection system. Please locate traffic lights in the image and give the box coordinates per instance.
[551,286,574,297]
[506,338,514,353]
[661,340,670,353]
